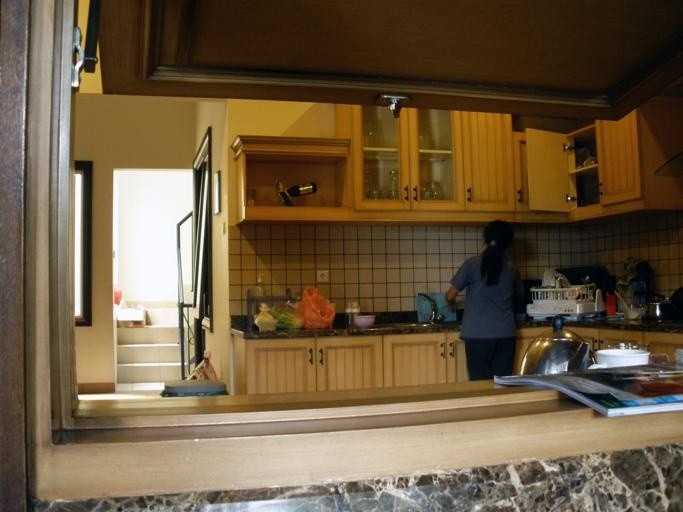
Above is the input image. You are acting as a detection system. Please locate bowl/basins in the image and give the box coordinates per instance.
[354,315,376,329]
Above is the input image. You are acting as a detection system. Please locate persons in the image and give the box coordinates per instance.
[443,219,528,382]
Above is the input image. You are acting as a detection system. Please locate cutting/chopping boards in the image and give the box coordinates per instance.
[415,291,458,324]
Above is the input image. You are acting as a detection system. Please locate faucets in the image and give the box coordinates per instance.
[417,292,438,317]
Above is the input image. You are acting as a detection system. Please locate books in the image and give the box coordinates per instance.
[492,359,683,419]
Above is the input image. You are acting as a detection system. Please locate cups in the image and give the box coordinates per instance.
[344,300,361,333]
[587,347,650,369]
[420,181,444,200]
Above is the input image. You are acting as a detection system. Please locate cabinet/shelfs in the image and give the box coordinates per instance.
[335,103,465,223]
[512,328,553,375]
[567,327,643,352]
[643,331,683,361]
[461,111,515,222]
[513,132,567,223]
[231,135,352,224]
[568,97,683,223]
[382,332,469,388]
[233,337,383,396]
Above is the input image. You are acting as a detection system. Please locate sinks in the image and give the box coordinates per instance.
[367,324,426,329]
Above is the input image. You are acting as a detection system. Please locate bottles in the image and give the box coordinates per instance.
[278,183,316,199]
[389,171,400,200]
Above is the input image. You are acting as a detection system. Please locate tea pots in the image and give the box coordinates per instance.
[519,316,594,377]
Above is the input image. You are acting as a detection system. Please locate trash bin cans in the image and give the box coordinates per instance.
[162,380,227,396]
